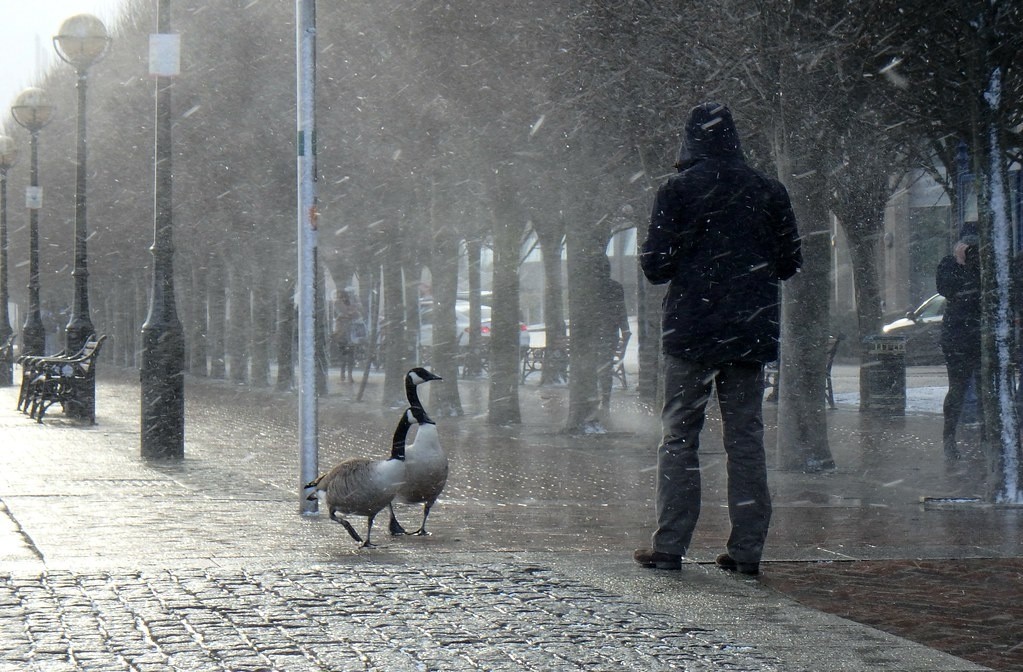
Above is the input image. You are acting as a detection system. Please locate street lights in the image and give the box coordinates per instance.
[10,86,58,361]
[53,12,112,426]
[0,133,19,388]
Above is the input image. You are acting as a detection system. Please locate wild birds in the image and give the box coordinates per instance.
[302,368,449,550]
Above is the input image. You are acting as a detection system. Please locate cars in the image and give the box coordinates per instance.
[882,293,952,350]
[383,300,531,361]
[393,289,524,331]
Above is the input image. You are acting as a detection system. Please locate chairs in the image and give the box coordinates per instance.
[418,331,631,389]
[18,348,68,414]
[0,332,18,386]
[29,333,108,426]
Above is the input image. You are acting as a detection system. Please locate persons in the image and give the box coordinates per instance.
[634,97,805,574]
[935,221,989,463]
[597,254,632,421]
[333,290,363,387]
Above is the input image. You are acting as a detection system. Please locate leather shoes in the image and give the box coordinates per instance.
[716,554,760,571]
[633,550,684,569]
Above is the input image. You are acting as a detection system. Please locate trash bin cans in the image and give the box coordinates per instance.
[864,334,908,415]
